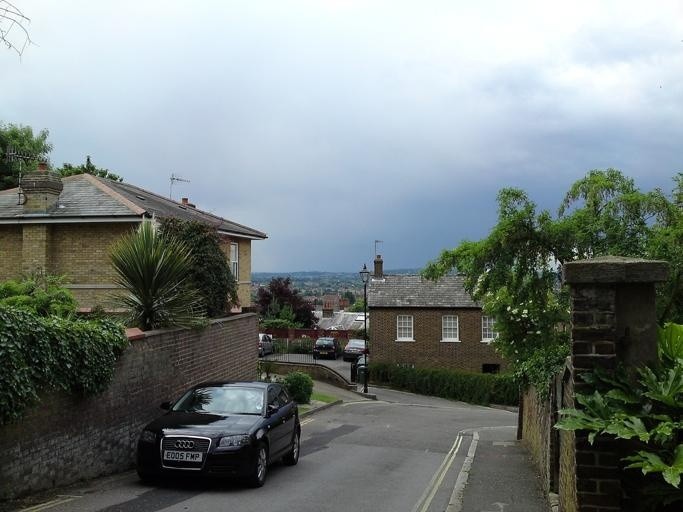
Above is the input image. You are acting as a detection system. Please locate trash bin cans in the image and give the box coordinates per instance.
[356,358,368,384]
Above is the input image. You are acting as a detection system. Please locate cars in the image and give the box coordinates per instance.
[343,339,368,361]
[136,381,302,489]
[313,338,341,360]
[259,333,275,357]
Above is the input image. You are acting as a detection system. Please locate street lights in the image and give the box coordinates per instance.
[359,265,369,393]
